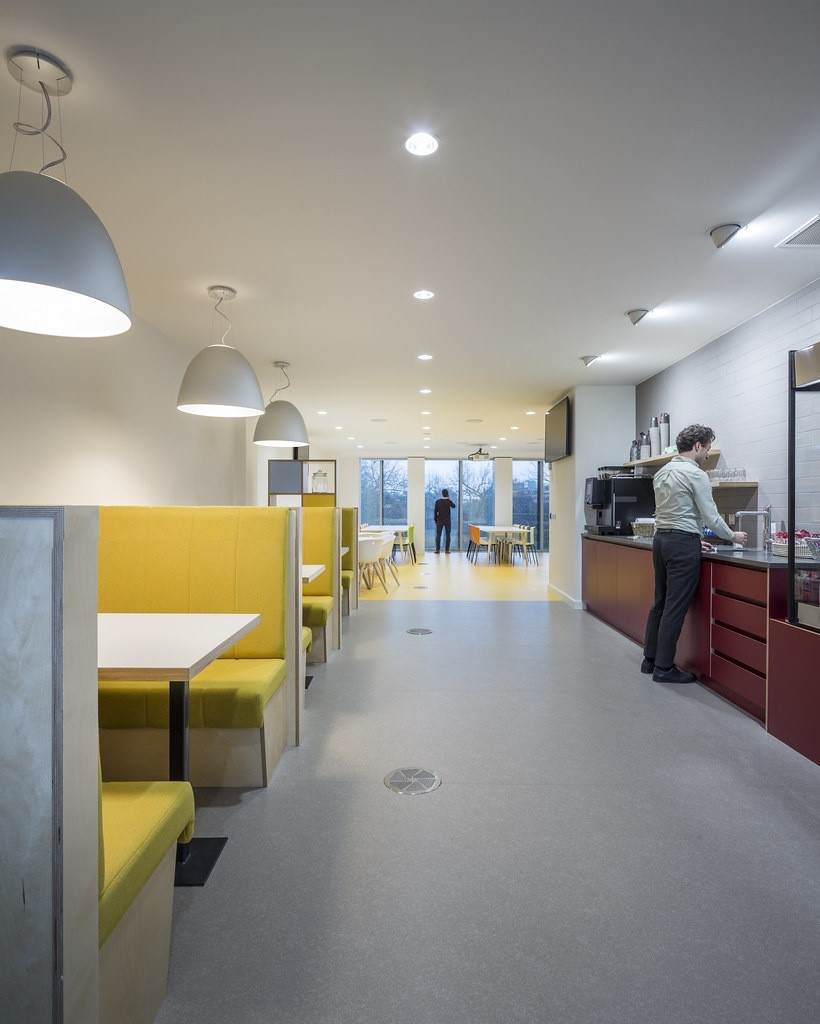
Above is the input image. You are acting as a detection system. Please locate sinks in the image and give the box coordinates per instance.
[708,545,762,552]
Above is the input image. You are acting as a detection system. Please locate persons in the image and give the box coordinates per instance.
[434,489,456,554]
[640,423,747,684]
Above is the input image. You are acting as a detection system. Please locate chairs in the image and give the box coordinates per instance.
[466,524,539,566]
[357,522,416,594]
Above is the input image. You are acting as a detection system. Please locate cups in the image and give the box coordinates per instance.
[706,467,746,484]
[733,531,744,549]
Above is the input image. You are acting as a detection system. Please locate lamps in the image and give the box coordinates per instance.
[711,224,741,249]
[582,357,597,367]
[628,310,648,325]
[253,362,309,448]
[177,286,267,419]
[0,52,132,338]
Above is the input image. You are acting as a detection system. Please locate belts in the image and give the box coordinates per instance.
[656,529,701,538]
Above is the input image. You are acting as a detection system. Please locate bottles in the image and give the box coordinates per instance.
[311,469,328,493]
[630,413,669,461]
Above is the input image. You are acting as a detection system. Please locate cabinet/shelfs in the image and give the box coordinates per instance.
[268,459,336,507]
[581,538,820,766]
[624,450,759,488]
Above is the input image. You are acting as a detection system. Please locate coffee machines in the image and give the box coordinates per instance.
[584,476,654,535]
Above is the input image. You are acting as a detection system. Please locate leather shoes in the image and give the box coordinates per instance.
[640,660,698,683]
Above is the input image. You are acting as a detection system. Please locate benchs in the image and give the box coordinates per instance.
[1,505,360,1024]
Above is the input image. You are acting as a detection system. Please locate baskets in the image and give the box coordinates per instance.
[630,522,656,537]
[772,543,814,559]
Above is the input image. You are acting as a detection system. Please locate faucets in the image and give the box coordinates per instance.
[734,504,772,550]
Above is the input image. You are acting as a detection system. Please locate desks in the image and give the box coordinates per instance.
[301,565,325,691]
[475,526,531,566]
[360,525,410,565]
[97,611,261,887]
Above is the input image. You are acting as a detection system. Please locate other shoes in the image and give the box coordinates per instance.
[434,550,440,554]
[445,550,451,554]
